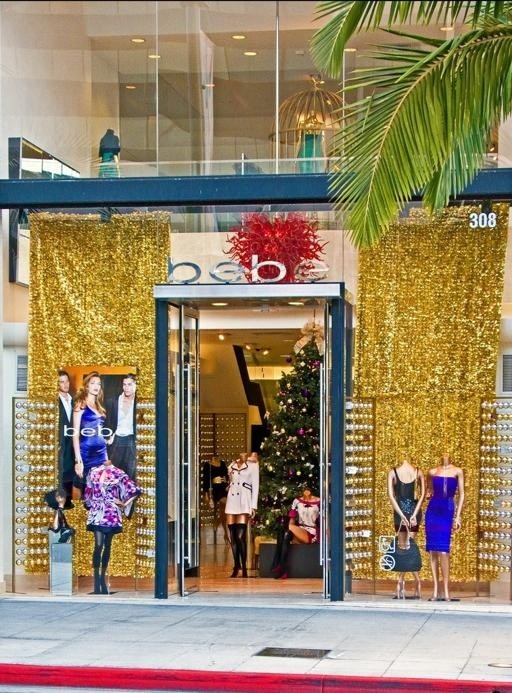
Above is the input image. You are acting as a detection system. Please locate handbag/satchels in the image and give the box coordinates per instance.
[316,527,330,544]
[203,493,215,509]
[384,536,421,572]
[396,496,422,525]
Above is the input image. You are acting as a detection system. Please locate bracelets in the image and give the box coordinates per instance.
[74,459,83,465]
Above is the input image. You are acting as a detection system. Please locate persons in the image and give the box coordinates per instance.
[425,455,465,604]
[223,452,259,577]
[270,488,321,581]
[84,459,141,594]
[246,451,260,570]
[59,371,76,496]
[203,455,230,544]
[72,372,109,497]
[104,373,136,480]
[387,459,426,599]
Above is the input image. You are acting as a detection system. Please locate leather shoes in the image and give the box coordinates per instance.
[269,564,281,573]
[276,572,289,580]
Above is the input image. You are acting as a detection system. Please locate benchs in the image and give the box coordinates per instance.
[257,542,325,579]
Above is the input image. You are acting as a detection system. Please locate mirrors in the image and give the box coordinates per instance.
[8,137,81,288]
